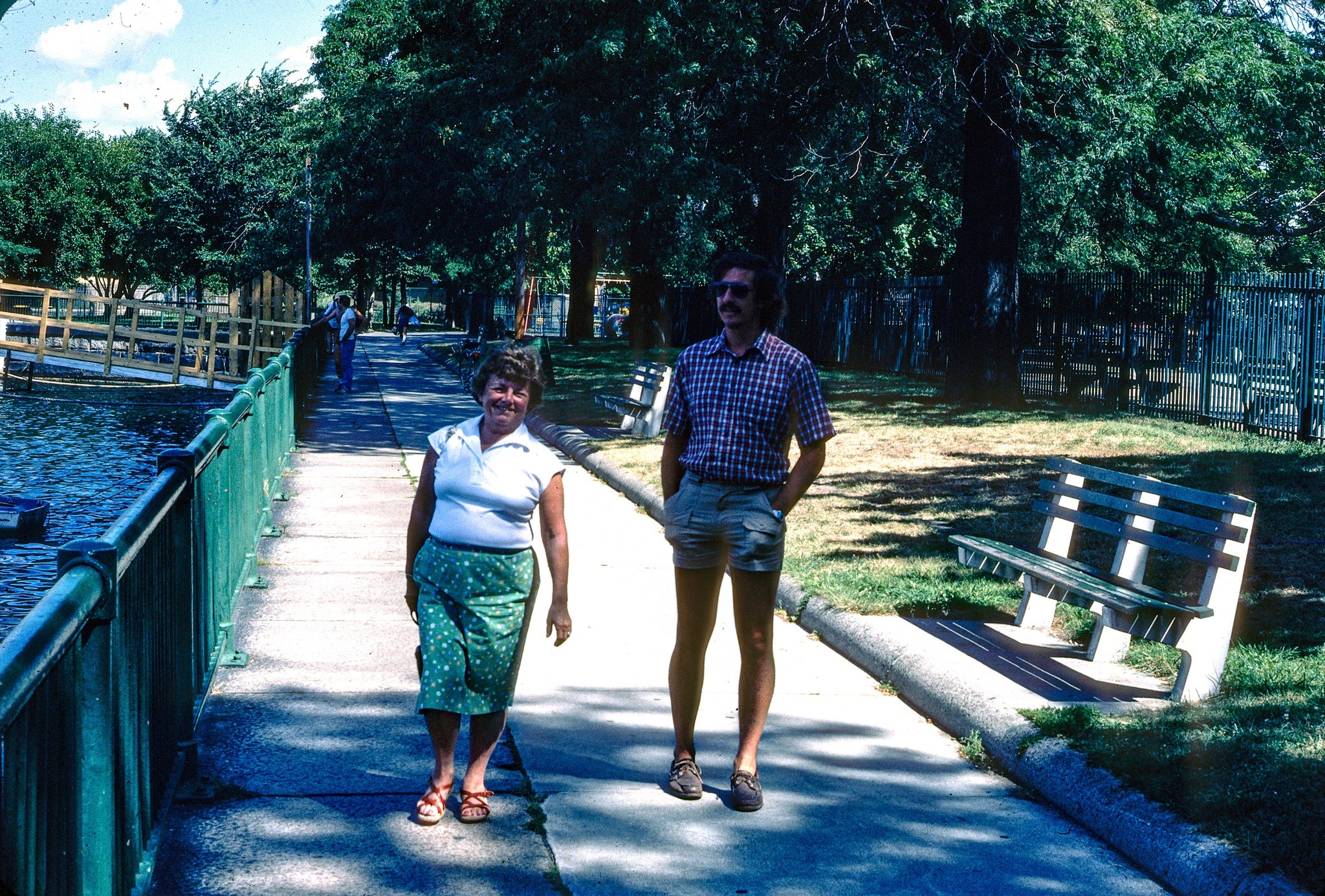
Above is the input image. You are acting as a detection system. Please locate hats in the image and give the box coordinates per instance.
[334,291,347,300]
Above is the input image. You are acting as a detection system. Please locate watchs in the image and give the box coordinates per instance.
[406,574,413,582]
[772,510,785,521]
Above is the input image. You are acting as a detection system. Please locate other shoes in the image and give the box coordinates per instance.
[668,755,704,799]
[729,766,765,811]
[335,384,342,391]
[401,342,404,346]
[336,388,351,394]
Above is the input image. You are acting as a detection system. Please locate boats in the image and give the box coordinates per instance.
[0,496,51,537]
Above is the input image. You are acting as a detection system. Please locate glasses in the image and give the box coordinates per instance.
[713,281,750,300]
[337,302,342,304]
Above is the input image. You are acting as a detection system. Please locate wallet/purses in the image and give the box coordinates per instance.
[415,645,424,682]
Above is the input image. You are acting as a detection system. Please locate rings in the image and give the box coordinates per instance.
[568,632,570,636]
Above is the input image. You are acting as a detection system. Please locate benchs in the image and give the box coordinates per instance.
[465,325,487,354]
[595,361,673,438]
[1056,341,1181,405]
[1230,347,1325,439]
[948,456,1257,701]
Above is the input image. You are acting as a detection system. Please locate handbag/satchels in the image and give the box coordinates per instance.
[409,314,421,327]
[392,327,400,336]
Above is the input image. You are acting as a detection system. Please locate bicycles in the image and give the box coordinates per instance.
[451,339,480,393]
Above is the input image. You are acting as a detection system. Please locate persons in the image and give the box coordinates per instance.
[495,316,505,340]
[406,349,572,824]
[660,250,838,811]
[311,291,367,395]
[395,297,414,346]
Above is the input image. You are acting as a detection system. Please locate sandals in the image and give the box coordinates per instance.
[417,776,455,826]
[458,788,494,820]
